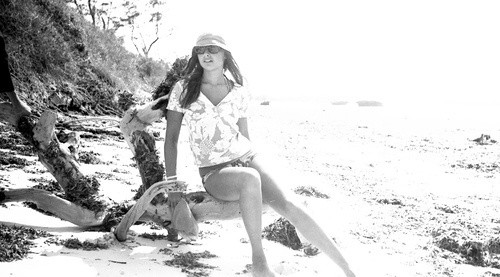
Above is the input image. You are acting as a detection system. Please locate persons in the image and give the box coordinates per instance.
[162,33,360,277]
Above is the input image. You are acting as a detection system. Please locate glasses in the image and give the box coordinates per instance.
[193,45,224,54]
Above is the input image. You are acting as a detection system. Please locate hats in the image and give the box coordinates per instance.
[195,34,230,51]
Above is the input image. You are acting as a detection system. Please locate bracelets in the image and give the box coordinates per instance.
[165,175,178,179]
